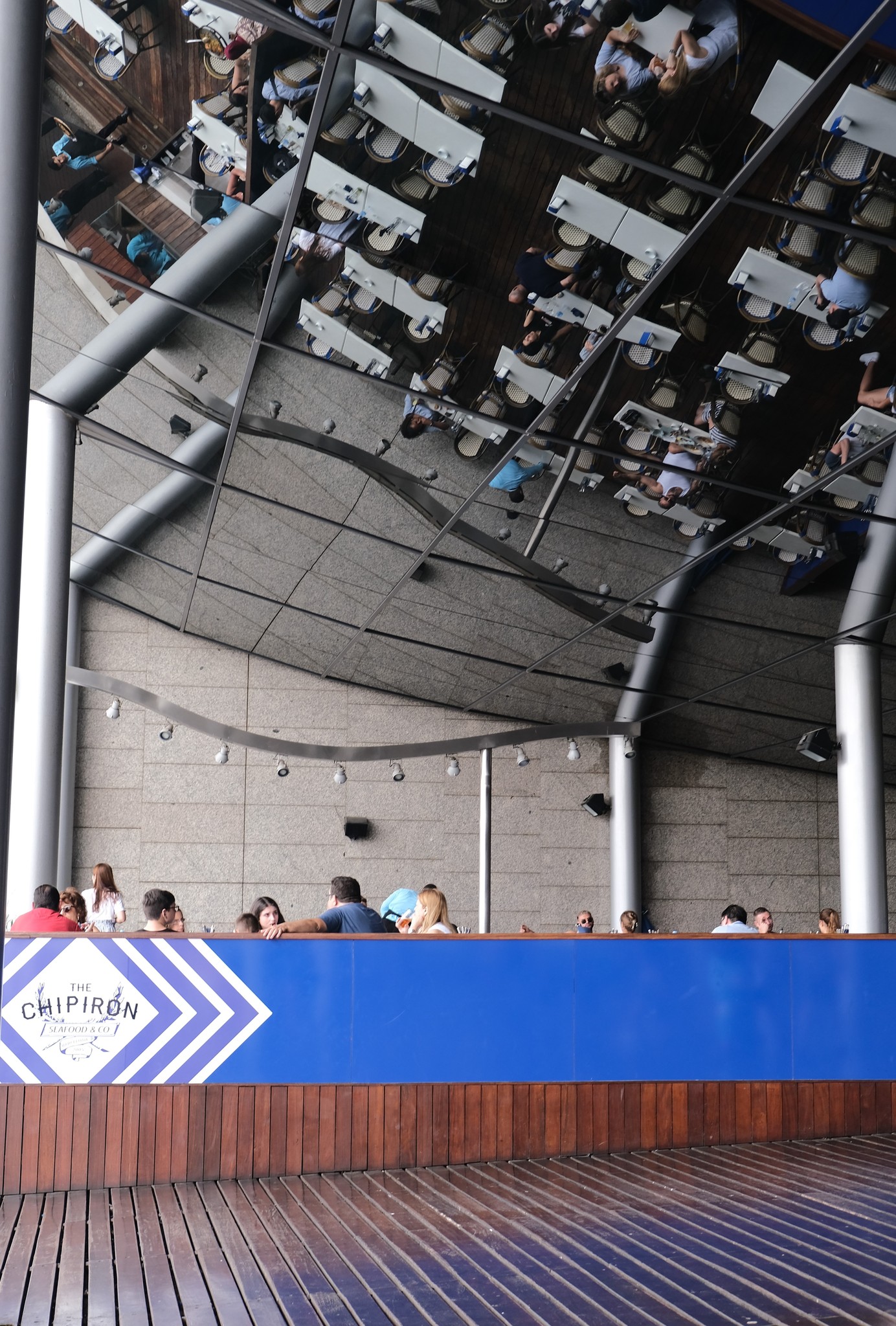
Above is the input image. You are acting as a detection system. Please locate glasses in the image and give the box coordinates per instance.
[173,918,185,924]
[719,917,725,926]
[581,917,593,924]
[664,497,669,501]
[324,893,337,901]
[362,898,367,903]
[169,905,179,912]
[59,904,75,913]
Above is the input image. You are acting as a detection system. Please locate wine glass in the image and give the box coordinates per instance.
[638,419,647,432]
[874,428,887,443]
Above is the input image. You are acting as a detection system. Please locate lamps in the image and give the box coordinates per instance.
[105,713,870,819]
[73,242,661,647]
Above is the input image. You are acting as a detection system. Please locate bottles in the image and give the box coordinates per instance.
[554,305,567,318]
[787,288,800,309]
[861,425,876,447]
[278,132,296,148]
[357,206,371,220]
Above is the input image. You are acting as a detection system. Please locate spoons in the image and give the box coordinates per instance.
[185,37,211,43]
[656,419,662,428]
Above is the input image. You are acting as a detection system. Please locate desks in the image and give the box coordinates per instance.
[184,0,245,47]
[188,101,248,174]
[268,0,896,615]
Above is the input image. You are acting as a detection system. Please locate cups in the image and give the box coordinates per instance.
[366,214,378,224]
[398,909,414,927]
[274,123,294,137]
[287,145,301,158]
[102,33,116,46]
[322,188,336,202]
[859,433,871,445]
[348,187,363,203]
[386,216,403,233]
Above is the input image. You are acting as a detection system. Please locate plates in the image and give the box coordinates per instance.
[445,407,455,414]
[677,436,718,455]
[430,403,441,411]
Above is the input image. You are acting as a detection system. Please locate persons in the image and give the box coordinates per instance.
[45,0,896,518]
[11,862,841,935]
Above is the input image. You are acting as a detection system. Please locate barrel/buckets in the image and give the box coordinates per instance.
[129,164,151,184]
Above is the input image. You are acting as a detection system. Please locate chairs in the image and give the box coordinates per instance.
[0,0,896,565]
